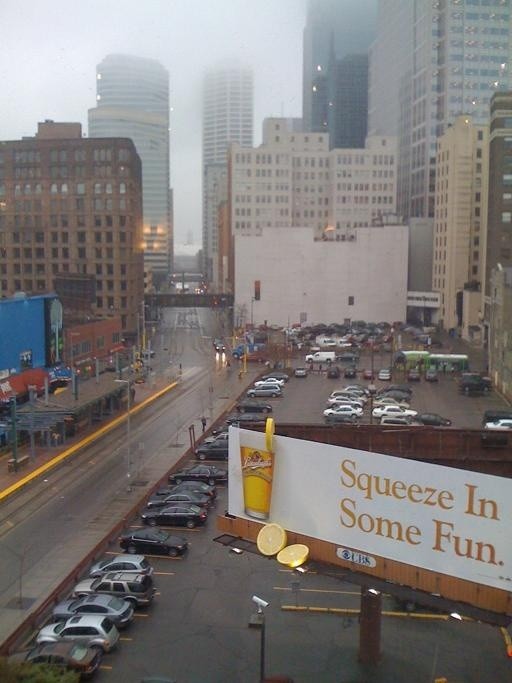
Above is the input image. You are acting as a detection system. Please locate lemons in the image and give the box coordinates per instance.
[276,544,309,569]
[256,524,287,557]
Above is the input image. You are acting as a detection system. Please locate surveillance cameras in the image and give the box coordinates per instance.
[250,595,270,613]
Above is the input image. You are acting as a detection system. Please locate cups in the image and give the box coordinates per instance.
[236,429,276,521]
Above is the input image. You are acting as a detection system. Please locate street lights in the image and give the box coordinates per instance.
[142,300,149,349]
[115,378,132,491]
[236,314,248,373]
[248,613,265,682]
[136,311,145,346]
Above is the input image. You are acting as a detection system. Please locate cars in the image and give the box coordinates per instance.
[177,462,216,472]
[52,594,135,630]
[120,526,188,559]
[171,278,189,291]
[390,583,441,612]
[195,320,308,460]
[146,490,212,508]
[141,503,208,529]
[138,349,155,357]
[307,320,511,444]
[156,479,218,500]
[8,640,102,680]
[168,461,228,485]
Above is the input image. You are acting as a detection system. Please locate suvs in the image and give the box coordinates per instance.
[73,571,156,610]
[36,615,119,655]
[88,554,153,580]
